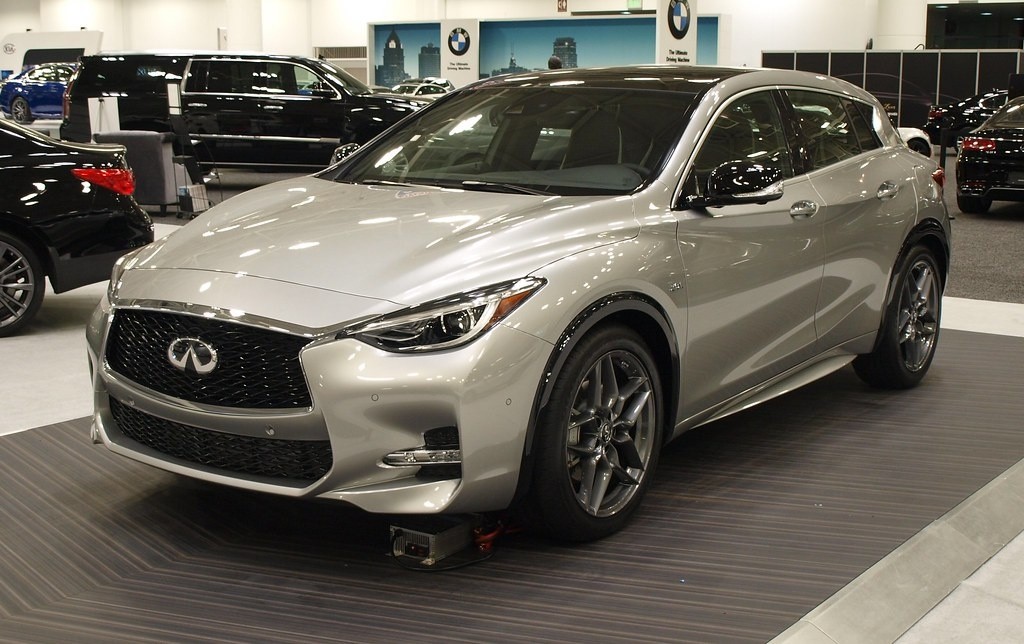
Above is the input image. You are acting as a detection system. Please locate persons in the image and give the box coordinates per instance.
[315,54,326,82]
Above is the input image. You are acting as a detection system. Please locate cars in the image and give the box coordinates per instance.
[82,62,959,544]
[892,124,935,158]
[366,74,456,102]
[0,61,83,126]
[0,118,156,339]
[920,74,1024,155]
[953,92,1024,215]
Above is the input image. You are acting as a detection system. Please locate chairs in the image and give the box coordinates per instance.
[545,110,623,190]
[640,111,754,197]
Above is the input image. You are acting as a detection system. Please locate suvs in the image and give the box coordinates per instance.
[55,52,434,188]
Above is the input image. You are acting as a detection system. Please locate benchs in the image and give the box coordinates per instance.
[94,130,211,217]
